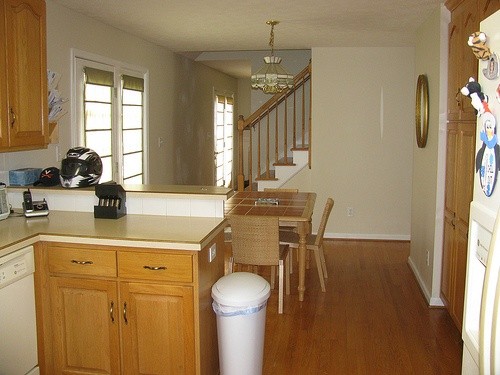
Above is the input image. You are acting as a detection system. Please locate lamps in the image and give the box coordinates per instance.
[250,21,294,94]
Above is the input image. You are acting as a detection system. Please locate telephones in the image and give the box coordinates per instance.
[22,188,49,218]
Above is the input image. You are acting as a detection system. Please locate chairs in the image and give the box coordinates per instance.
[225,214,291,315]
[270,197,335,291]
[263,188,299,274]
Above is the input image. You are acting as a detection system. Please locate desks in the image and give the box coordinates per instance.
[225,190,317,301]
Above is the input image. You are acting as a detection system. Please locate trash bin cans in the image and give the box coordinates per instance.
[211,272,271,375]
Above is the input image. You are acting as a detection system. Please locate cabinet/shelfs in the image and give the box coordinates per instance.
[31,229,225,375]
[0,0,50,154]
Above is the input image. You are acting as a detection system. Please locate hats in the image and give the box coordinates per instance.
[40,167,61,186]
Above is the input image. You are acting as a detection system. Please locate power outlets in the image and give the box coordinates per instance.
[424,249,429,267]
[347,207,353,217]
[209,242,216,262]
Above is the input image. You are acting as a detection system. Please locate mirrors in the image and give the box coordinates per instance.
[415,75,429,147]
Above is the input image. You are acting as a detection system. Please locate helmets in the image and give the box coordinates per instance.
[59,146,103,188]
